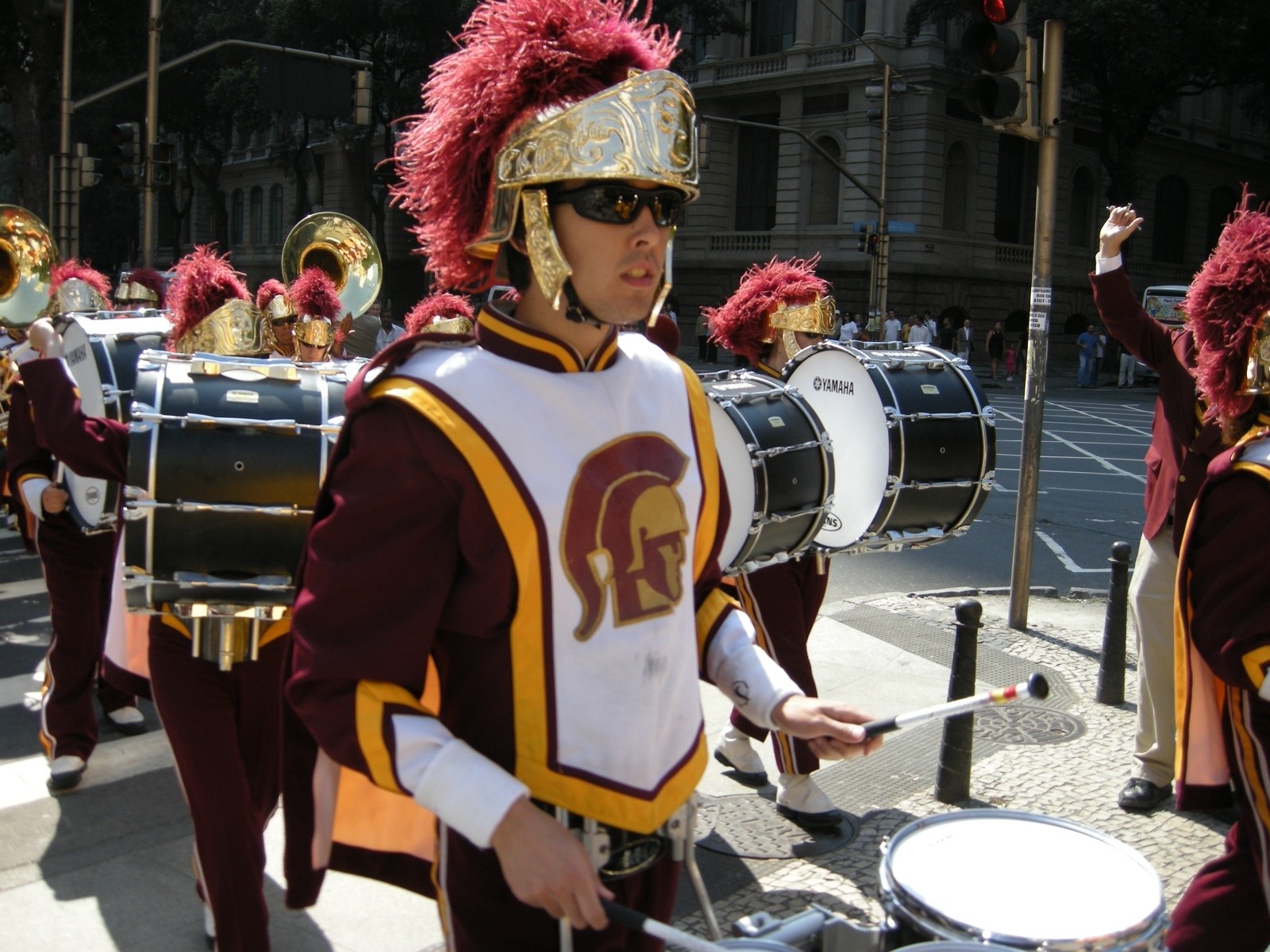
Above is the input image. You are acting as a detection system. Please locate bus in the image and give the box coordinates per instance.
[1132,285,1192,390]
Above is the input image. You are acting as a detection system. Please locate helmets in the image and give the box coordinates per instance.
[159,240,278,360]
[1175,183,1270,432]
[287,266,343,361]
[370,0,708,328]
[257,276,298,328]
[42,259,115,319]
[697,250,836,360]
[402,291,478,338]
[114,267,167,310]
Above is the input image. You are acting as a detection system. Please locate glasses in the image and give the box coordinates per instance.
[301,340,328,349]
[128,298,148,305]
[547,180,685,228]
[804,331,830,339]
[856,316,860,318]
[271,315,298,326]
[113,298,130,306]
[844,314,850,317]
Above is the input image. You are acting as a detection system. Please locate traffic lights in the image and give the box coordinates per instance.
[40,0,65,19]
[81,156,103,189]
[350,70,374,127]
[965,0,1029,128]
[869,232,880,254]
[113,121,140,188]
[150,142,175,186]
[857,223,873,253]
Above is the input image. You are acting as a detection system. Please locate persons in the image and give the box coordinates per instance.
[985,321,1017,382]
[29,244,290,952]
[658,300,720,366]
[1076,324,1105,388]
[289,1,891,952]
[617,320,646,336]
[1091,204,1270,952]
[6,261,147,789]
[1117,341,1136,388]
[828,310,972,366]
[705,258,844,829]
[113,269,163,319]
[365,301,405,357]
[257,273,355,362]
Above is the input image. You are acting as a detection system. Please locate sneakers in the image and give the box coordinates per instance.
[1116,777,1174,810]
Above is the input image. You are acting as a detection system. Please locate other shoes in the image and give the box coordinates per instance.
[775,772,844,830]
[45,754,89,797]
[707,362,714,365]
[101,705,147,736]
[713,720,769,787]
[993,376,997,381]
[197,899,219,951]
[1006,376,1013,381]
[698,358,704,362]
[7,513,20,532]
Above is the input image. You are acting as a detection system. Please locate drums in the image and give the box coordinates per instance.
[681,368,836,573]
[51,310,178,536]
[778,336,1001,552]
[878,806,1167,952]
[119,348,356,624]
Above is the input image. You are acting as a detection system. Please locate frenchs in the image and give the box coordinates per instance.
[282,211,384,320]
[0,204,63,329]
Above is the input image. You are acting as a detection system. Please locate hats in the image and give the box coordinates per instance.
[835,310,841,315]
